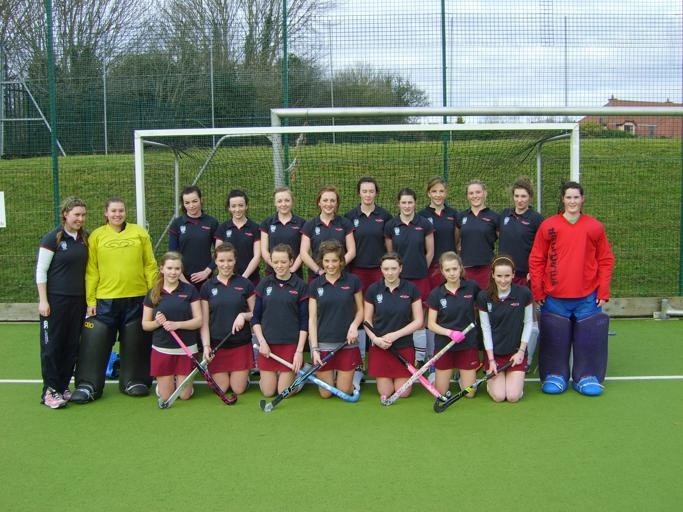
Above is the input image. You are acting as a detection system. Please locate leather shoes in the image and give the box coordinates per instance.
[449,330,466,343]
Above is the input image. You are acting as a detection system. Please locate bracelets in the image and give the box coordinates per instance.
[519,349,525,352]
[311,348,321,352]
[316,267,321,275]
[489,359,495,361]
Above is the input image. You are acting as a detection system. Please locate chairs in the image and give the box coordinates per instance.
[541,370,568,393]
[572,375,605,396]
[121,379,149,396]
[71,380,96,404]
[43,386,72,409]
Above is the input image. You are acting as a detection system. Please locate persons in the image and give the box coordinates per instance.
[251,243,309,398]
[258,187,307,280]
[344,176,394,373]
[476,256,534,401]
[496,178,545,374]
[199,242,256,395]
[526,180,616,396]
[427,251,482,398]
[364,252,424,398]
[299,184,356,284]
[34,194,90,409]
[384,188,437,373]
[214,188,262,375]
[455,179,500,373]
[69,195,160,406]
[308,240,364,398]
[169,184,220,369]
[141,251,203,402]
[417,176,460,290]
